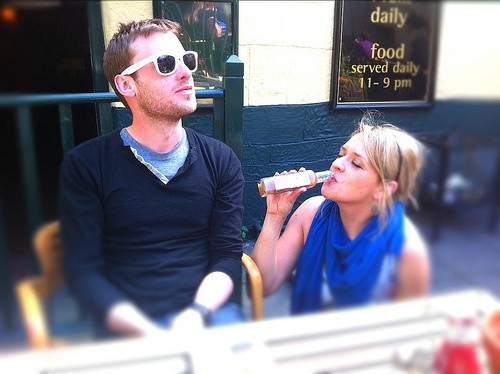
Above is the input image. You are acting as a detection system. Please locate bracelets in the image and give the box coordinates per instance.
[192,302,211,326]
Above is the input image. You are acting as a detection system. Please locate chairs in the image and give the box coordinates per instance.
[16,221,263,351]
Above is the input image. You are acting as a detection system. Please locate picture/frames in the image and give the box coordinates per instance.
[330,0,440,110]
[152,0,239,87]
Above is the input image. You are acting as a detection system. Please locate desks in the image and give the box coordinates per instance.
[0,289,500,374]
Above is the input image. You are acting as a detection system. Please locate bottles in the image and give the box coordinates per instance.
[258,169,333,198]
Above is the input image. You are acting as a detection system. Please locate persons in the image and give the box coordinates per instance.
[246,124,428,316]
[58,19,244,343]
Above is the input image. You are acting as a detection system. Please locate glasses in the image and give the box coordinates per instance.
[121,51,198,76]
